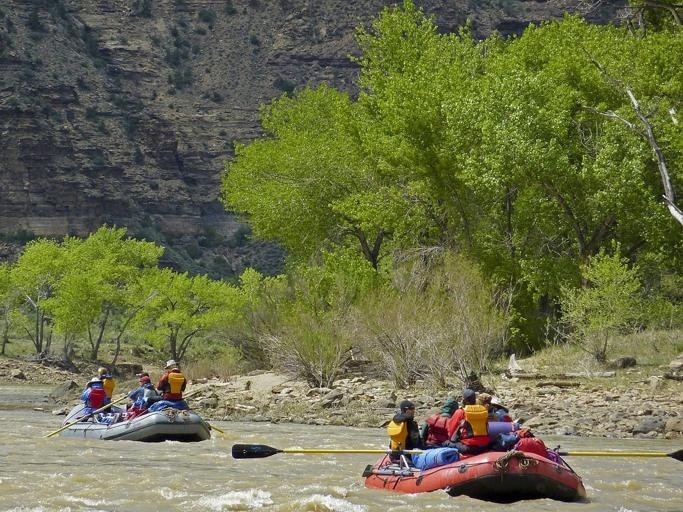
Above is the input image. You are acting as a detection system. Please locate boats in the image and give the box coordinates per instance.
[56,402,214,443]
[360,440,589,505]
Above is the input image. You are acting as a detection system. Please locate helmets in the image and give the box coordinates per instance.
[98,368,108,374]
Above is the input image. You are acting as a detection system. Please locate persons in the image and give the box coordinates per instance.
[389,389,508,463]
[80,360,187,422]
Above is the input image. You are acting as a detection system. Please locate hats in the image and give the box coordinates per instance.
[88,377,103,383]
[464,388,476,400]
[444,399,459,410]
[140,376,150,383]
[401,401,413,408]
[479,392,492,402]
[136,372,149,377]
[164,360,179,369]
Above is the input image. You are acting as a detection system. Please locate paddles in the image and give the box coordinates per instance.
[559,450,683,462]
[233,444,421,458]
